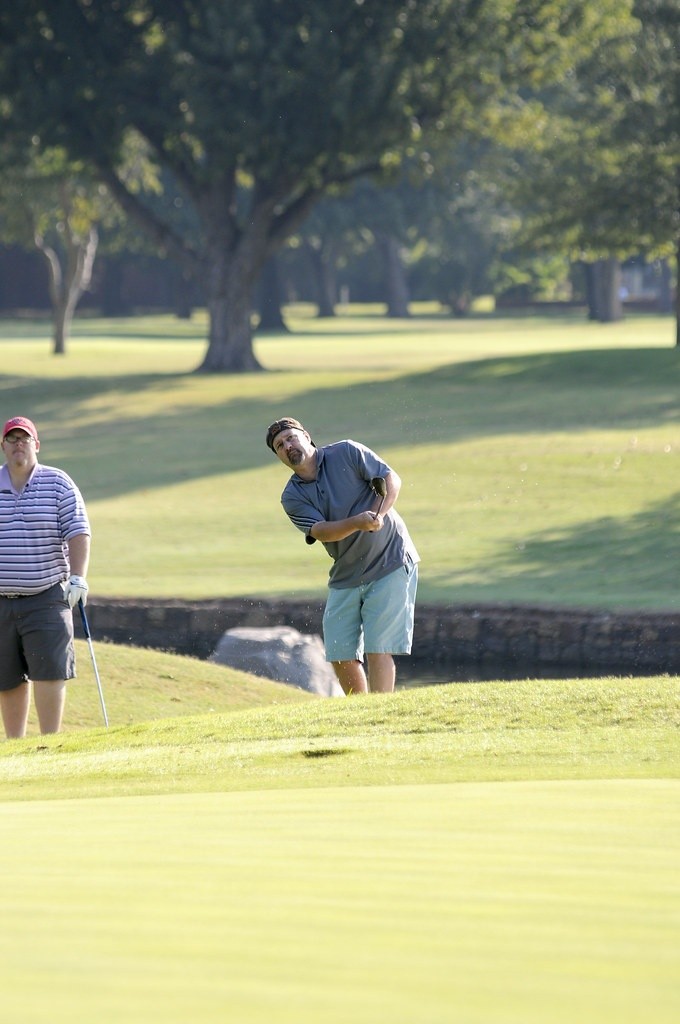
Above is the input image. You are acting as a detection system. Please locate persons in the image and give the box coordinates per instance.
[0,417,92,741]
[266,416,421,694]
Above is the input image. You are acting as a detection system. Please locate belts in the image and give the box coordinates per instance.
[1,596,26,599]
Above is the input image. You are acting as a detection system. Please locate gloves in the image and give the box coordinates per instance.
[64,574,88,608]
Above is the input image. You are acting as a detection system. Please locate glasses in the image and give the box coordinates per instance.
[5,434,31,443]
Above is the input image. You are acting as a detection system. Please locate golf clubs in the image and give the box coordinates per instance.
[367,477,388,533]
[77,598,109,729]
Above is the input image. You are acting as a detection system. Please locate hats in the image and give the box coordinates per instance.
[267,417,316,454]
[3,416,36,441]
